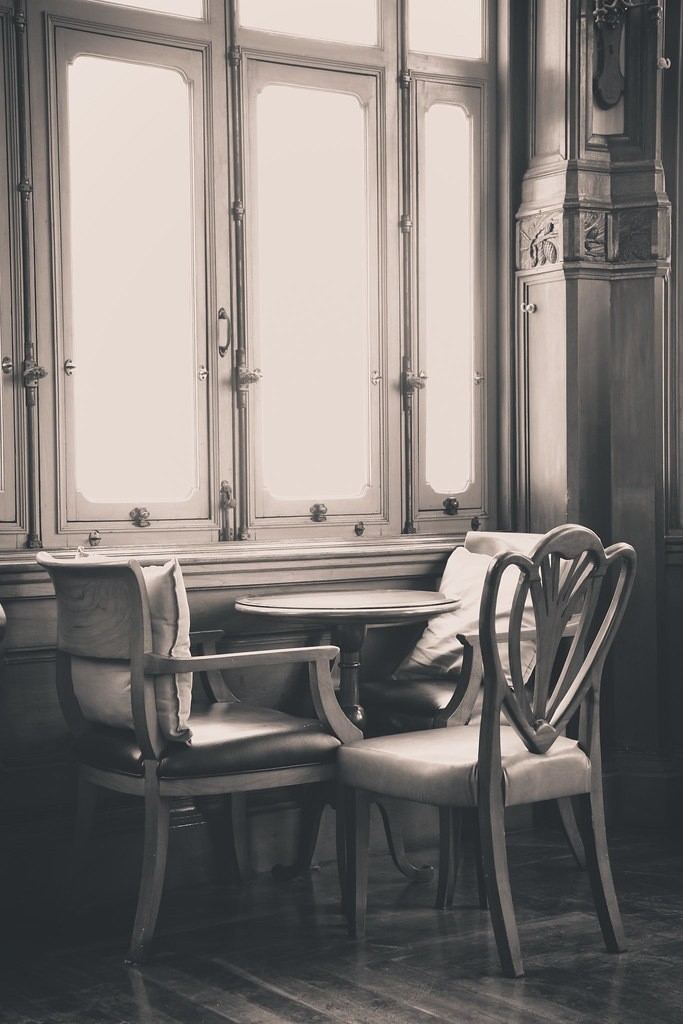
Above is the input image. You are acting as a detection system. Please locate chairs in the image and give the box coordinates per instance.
[37,551,368,964]
[339,522,641,977]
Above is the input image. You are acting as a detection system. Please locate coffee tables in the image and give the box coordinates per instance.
[236,588,464,882]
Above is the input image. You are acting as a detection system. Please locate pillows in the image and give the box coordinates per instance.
[391,546,569,693]
[69,545,193,746]
